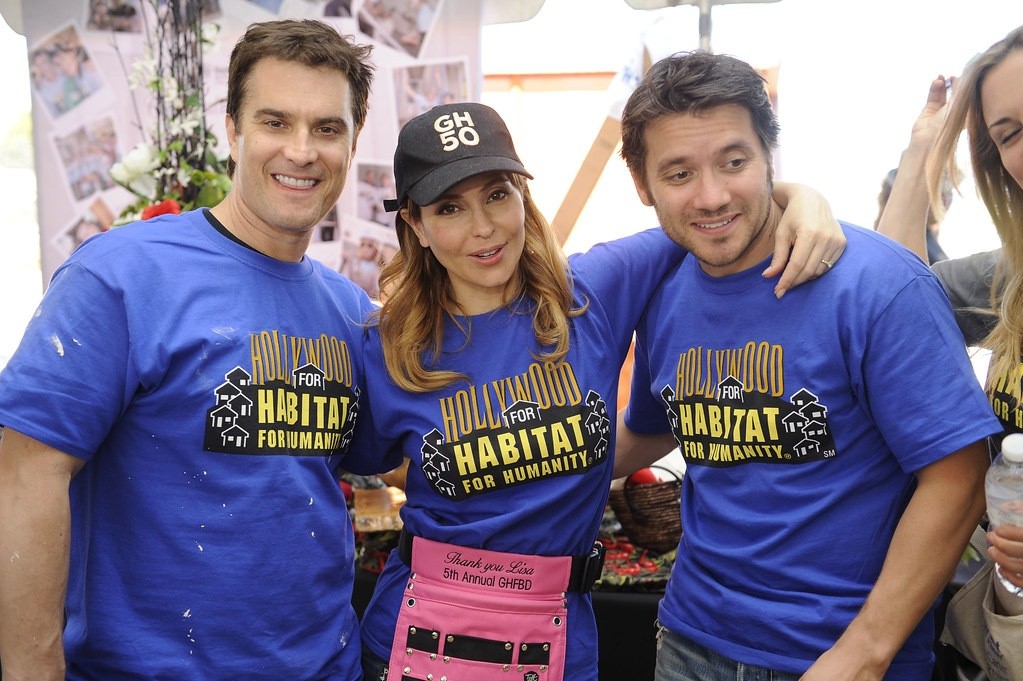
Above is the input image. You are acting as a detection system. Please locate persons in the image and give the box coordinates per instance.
[359,166,395,227]
[875,25,1023,680]
[322,102,847,681]
[360,0,433,53]
[377,51,1007,681]
[0,16,409,681]
[402,82,454,115]
[30,47,100,117]
[876,164,950,264]
[338,236,399,302]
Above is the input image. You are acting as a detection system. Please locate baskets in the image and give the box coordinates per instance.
[609,465,682,551]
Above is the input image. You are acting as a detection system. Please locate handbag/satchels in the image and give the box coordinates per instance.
[386,527,607,681]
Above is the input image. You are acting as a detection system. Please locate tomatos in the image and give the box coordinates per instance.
[630,468,658,484]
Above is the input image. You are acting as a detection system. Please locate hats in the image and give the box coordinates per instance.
[383,102,533,212]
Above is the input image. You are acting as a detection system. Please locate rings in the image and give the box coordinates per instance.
[821,259,833,268]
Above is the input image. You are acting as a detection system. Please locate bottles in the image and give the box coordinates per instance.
[984,433,1023,598]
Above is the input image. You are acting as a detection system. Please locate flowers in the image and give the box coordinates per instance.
[107,0,235,228]
